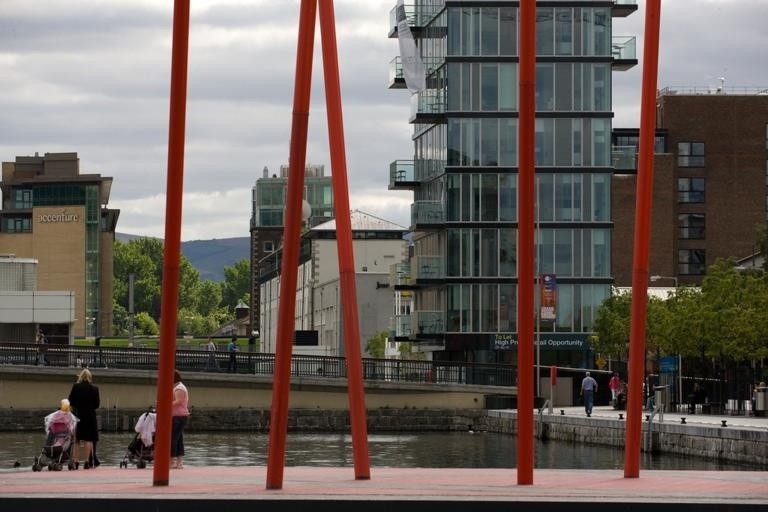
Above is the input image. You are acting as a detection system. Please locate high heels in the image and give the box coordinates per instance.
[75,460,89,469]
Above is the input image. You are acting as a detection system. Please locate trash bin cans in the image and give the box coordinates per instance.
[655,386,666,413]
[756,386,768,415]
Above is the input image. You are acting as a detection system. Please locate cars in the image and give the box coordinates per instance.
[70,358,108,368]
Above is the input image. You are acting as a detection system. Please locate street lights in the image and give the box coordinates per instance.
[649,274,683,404]
[734,266,765,277]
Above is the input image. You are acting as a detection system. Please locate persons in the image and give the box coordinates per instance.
[609,373,619,399]
[170,371,191,469]
[227,337,240,373]
[200,335,222,373]
[77,355,84,367]
[69,369,100,470]
[752,384,757,417]
[580,372,598,417]
[33,329,51,366]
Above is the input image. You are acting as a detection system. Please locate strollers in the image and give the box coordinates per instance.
[32,408,77,471]
[119,405,157,469]
